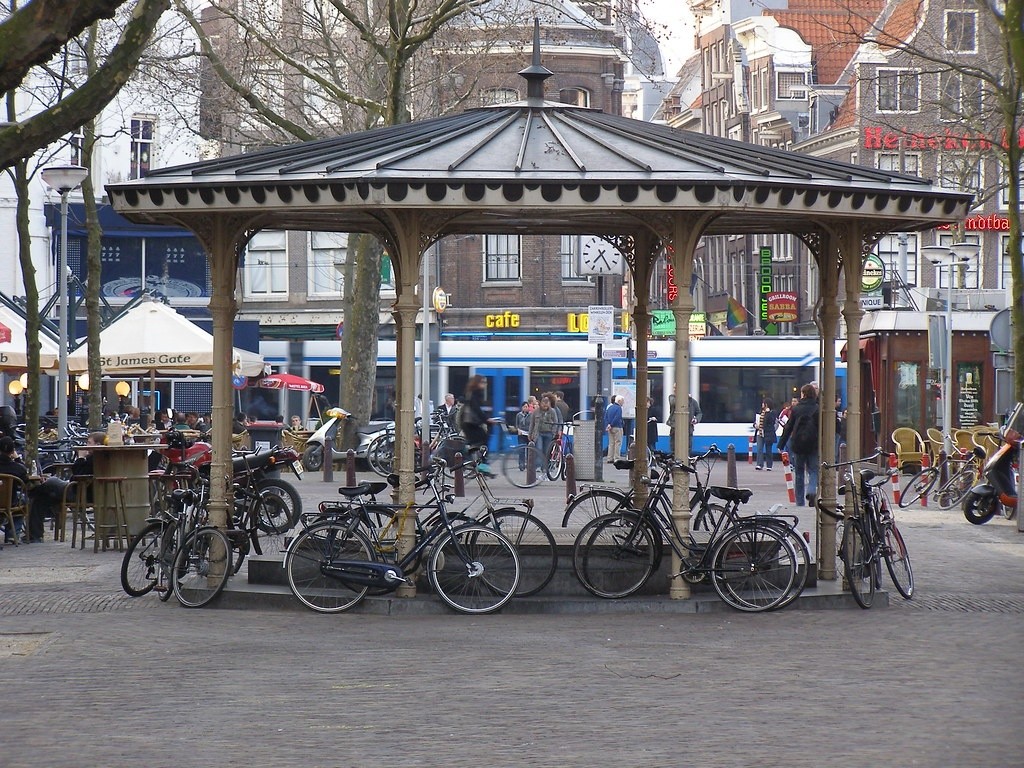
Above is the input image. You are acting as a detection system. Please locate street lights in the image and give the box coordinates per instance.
[78,373,90,411]
[8,379,23,415]
[20,372,28,418]
[919,242,981,479]
[115,381,130,414]
[40,162,90,465]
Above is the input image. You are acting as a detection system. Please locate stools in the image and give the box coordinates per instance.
[70,470,191,553]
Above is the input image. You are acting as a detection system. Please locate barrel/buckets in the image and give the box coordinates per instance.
[93,449,150,537]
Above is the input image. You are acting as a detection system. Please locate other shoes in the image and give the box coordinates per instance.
[808,494,815,507]
[21,535,44,543]
[8,535,21,544]
[755,466,762,470]
[767,468,772,471]
[607,460,615,463]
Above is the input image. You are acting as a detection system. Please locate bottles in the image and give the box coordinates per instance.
[32,460,37,476]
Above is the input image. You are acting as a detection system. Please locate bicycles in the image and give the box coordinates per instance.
[562,415,812,613]
[367,402,549,489]
[119,445,294,609]
[806,446,914,611]
[279,445,558,615]
[9,413,154,483]
[977,431,1016,520]
[897,435,986,512]
[544,420,580,482]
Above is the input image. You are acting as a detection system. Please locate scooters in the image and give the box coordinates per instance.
[302,406,396,472]
[962,432,1020,525]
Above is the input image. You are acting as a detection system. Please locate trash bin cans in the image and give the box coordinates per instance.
[570,410,603,482]
[244,421,285,496]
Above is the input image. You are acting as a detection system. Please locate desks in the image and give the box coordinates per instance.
[294,431,318,440]
[71,430,200,546]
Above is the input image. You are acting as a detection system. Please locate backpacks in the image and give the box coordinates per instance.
[792,404,819,455]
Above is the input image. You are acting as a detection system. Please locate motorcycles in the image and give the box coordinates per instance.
[148,406,305,536]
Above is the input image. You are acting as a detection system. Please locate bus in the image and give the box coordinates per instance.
[246,335,847,456]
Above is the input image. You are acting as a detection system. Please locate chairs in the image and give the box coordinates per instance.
[280,431,342,473]
[54,462,93,541]
[891,425,999,476]
[233,429,248,451]
[0,474,29,546]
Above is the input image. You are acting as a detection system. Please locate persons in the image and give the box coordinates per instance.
[834,396,848,468]
[436,393,464,442]
[46,408,58,427]
[78,395,89,426]
[516,391,570,472]
[777,382,819,506]
[274,415,308,432]
[153,408,212,442]
[102,396,151,430]
[463,374,500,478]
[605,395,625,463]
[233,414,257,435]
[0,432,107,543]
[648,398,658,469]
[665,382,703,481]
[753,398,779,471]
[778,396,799,471]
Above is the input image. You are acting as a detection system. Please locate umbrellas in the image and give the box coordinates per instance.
[232,371,324,418]
[0,292,273,422]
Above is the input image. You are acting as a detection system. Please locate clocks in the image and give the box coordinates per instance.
[582,237,621,272]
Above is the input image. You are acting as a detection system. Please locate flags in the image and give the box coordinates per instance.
[690,274,697,294]
[726,296,748,330]
